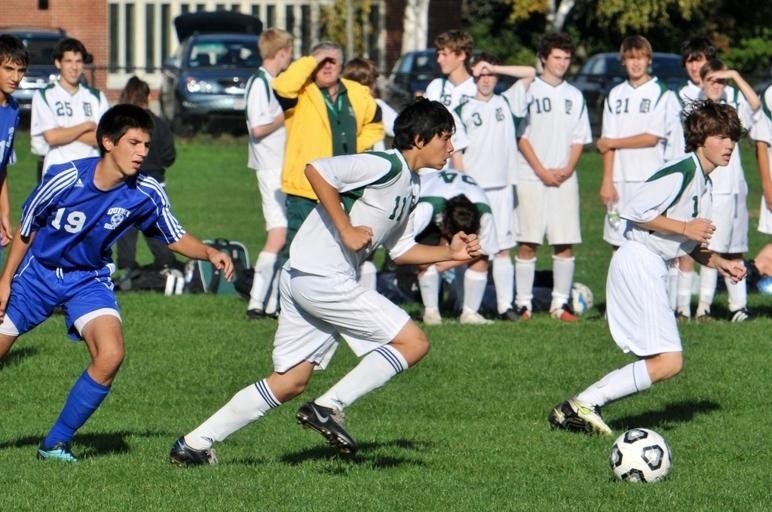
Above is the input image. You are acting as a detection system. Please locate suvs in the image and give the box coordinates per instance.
[157,11,267,131]
[0,25,96,120]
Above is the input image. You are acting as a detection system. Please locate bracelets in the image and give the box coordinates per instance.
[679,221,690,238]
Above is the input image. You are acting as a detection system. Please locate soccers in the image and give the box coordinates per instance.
[567,282,593,313]
[611,428,670,482]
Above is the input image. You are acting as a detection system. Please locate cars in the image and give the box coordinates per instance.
[567,52,688,136]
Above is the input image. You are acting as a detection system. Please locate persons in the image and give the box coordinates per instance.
[412,29,535,324]
[0,100,239,465]
[168,100,483,466]
[0,33,29,246]
[597,34,675,248]
[107,73,177,278]
[244,28,397,320]
[514,36,592,321]
[665,38,762,321]
[745,84,772,292]
[548,101,750,439]
[27,39,110,185]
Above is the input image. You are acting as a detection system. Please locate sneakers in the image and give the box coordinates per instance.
[423,306,579,325]
[676,309,752,322]
[296,399,355,454]
[37,439,78,461]
[170,435,218,467]
[548,399,611,433]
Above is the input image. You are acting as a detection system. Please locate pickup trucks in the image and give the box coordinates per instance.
[387,49,511,110]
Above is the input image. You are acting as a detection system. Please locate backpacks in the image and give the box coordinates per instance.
[182,238,248,294]
